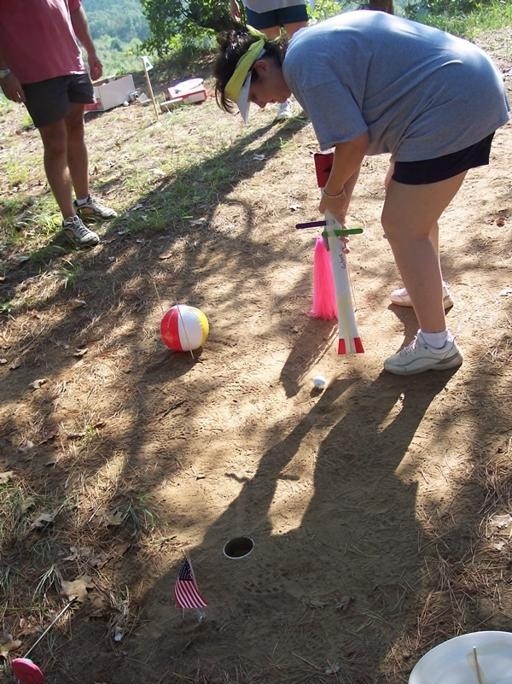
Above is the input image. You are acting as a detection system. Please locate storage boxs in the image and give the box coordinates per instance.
[167,85,206,105]
[84,75,135,112]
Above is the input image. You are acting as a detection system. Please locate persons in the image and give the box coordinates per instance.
[207,8,510,377]
[230,0,309,122]
[0,0,118,248]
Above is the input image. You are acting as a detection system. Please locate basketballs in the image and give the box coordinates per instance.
[161,304,209,352]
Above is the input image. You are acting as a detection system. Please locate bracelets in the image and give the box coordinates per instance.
[321,188,345,199]
[0,67,12,79]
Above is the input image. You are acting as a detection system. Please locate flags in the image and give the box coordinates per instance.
[174,557,209,608]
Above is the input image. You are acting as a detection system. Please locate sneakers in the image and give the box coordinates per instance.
[74,197,117,221]
[61,218,101,248]
[277,99,291,121]
[391,283,452,310]
[385,332,463,375]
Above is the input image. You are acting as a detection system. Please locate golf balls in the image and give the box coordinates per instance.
[315,377,325,387]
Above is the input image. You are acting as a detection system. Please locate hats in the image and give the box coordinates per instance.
[231,45,263,124]
